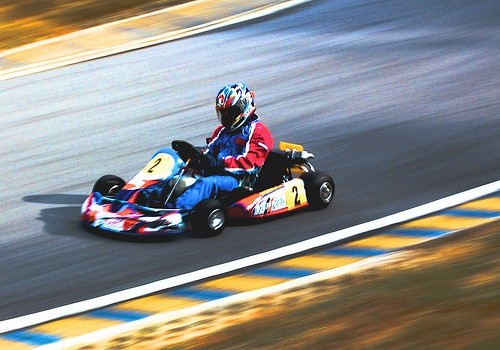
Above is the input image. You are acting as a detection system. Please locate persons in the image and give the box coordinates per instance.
[140,83,274,217]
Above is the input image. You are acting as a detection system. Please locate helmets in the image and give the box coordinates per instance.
[215,82,257,131]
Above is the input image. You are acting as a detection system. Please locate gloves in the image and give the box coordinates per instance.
[197,153,223,170]
